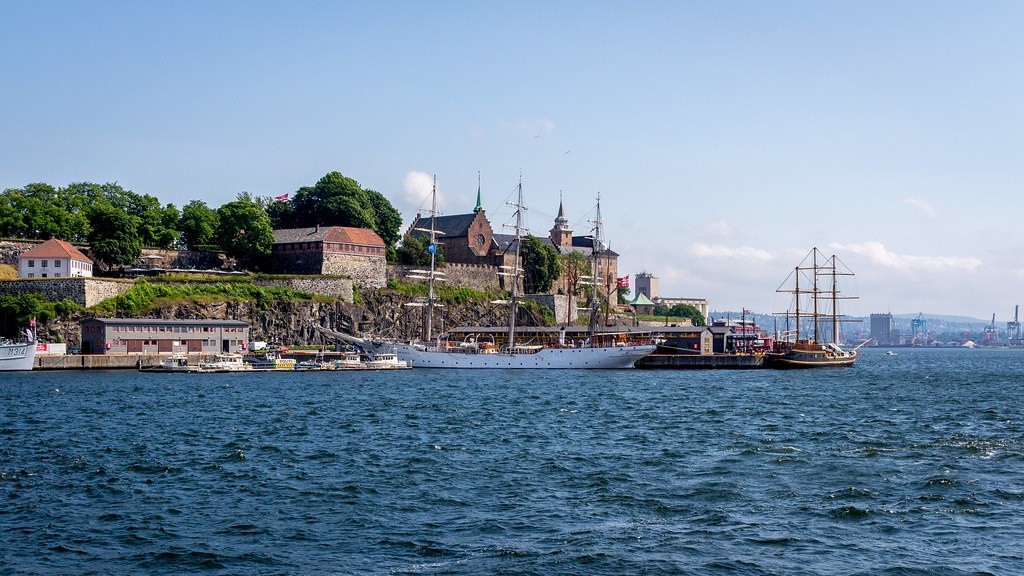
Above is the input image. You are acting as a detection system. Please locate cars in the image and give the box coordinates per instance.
[67,345,83,355]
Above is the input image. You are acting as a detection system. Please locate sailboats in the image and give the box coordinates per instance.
[770,245,873,366]
[311,173,659,370]
[727,307,777,355]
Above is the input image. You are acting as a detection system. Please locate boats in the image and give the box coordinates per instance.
[1,317,37,372]
[154,341,413,371]
[884,350,898,355]
